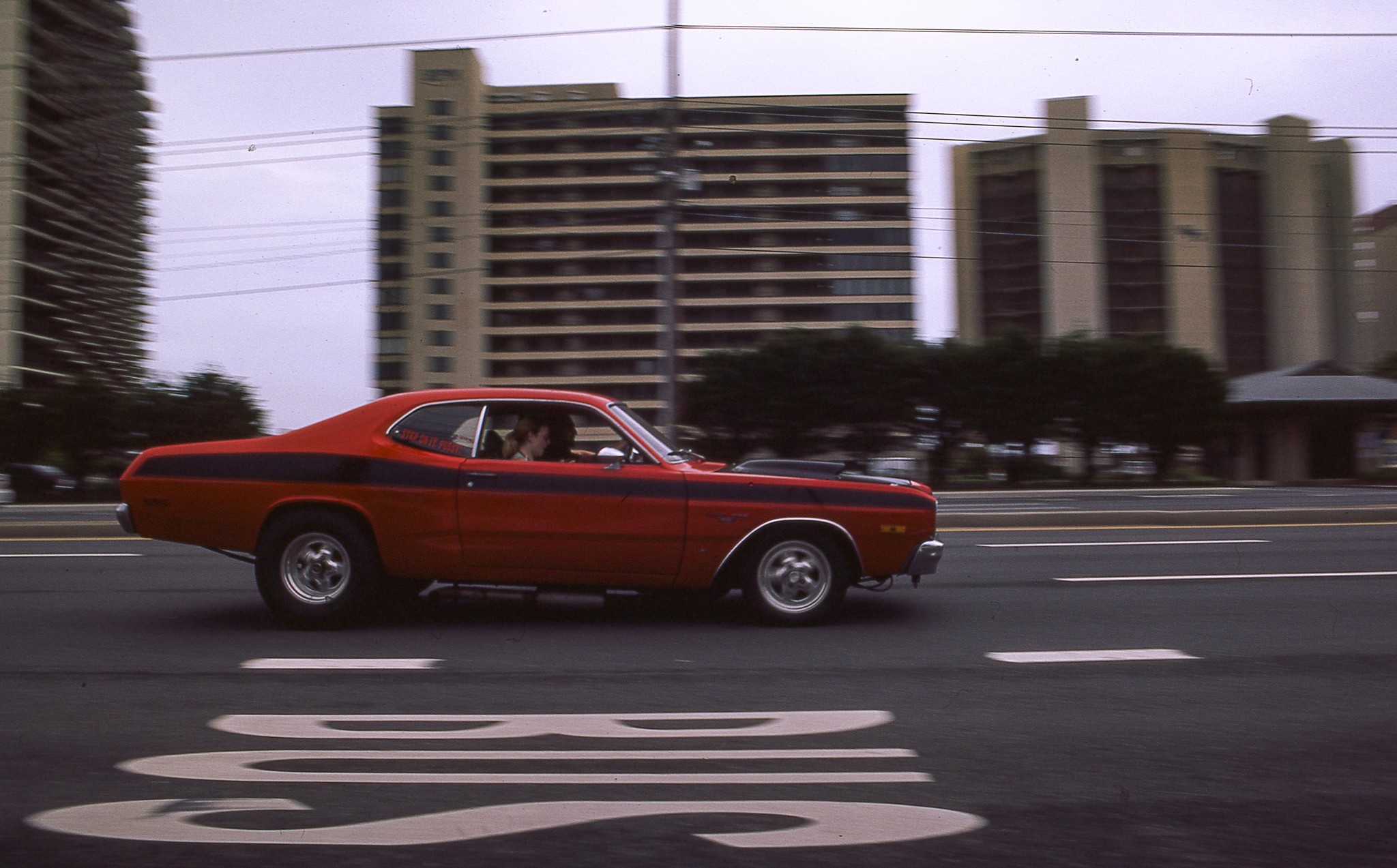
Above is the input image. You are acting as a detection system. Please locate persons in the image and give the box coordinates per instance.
[502,412,550,462]
[535,410,596,463]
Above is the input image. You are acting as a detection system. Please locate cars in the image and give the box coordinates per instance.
[112,386,947,629]
